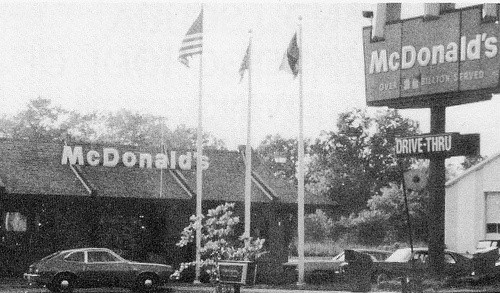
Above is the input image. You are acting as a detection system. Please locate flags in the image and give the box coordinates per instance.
[278,23,303,79]
[177,8,203,67]
[238,37,252,86]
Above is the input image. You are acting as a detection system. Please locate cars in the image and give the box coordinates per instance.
[294,250,385,285]
[369,247,472,285]
[23,248,175,293]
